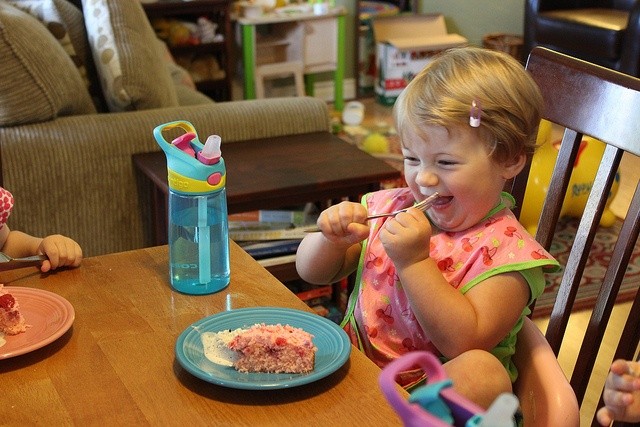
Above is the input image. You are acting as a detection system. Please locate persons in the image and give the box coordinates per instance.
[0,186,84,276]
[595,358,640,426]
[292,43,561,413]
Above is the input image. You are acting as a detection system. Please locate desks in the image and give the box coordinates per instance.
[231,6,347,111]
[132,131,401,324]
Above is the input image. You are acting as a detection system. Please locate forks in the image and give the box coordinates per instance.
[304,191,441,234]
[0,250,50,264]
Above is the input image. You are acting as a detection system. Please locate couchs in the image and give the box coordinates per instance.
[0,0,332,257]
[522,0,640,78]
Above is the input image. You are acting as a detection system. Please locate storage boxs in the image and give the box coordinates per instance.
[370,12,468,105]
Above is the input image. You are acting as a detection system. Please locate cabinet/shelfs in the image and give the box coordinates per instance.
[139,0,232,103]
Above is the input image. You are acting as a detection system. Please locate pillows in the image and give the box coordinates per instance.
[3,0,108,112]
[81,0,179,112]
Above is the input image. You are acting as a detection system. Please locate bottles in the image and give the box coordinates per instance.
[153,120,231,295]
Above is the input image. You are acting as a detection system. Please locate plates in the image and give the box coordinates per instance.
[176,306,352,392]
[1,283,76,361]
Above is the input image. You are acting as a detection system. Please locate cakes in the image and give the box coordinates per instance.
[0,283,29,336]
[200,322,315,374]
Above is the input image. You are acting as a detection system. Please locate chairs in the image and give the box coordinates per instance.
[502,44,640,426]
